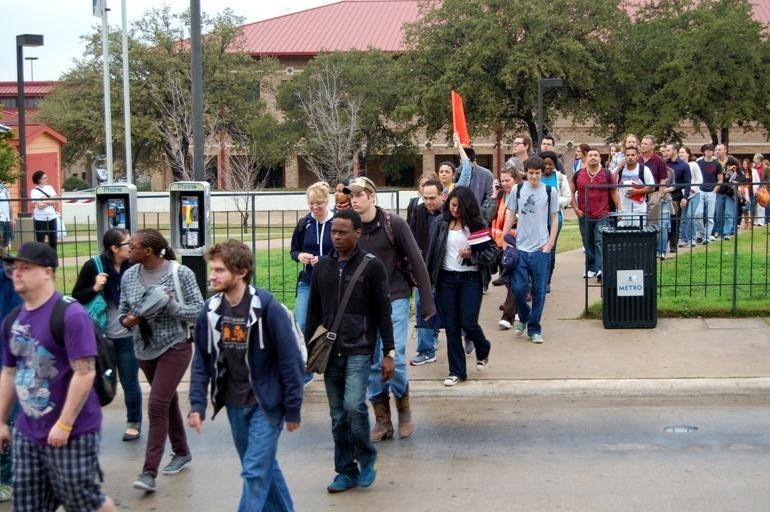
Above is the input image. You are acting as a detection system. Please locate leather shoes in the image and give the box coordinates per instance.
[122,431,139,440]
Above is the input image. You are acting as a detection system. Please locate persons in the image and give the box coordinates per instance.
[0,221,24,502]
[343,176,438,444]
[304,208,396,493]
[0,179,16,251]
[330,179,352,214]
[185,239,305,512]
[289,181,336,389]
[116,227,206,492]
[0,241,116,511]
[407,134,572,387]
[72,227,143,441]
[30,170,60,282]
[569,134,770,280]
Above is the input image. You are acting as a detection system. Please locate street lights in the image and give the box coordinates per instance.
[25,57,39,81]
[538,77,563,154]
[13,34,44,250]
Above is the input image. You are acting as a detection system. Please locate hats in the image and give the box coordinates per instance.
[4,242,58,267]
[703,144,715,151]
[343,177,376,194]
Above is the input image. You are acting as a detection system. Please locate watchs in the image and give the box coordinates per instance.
[384,349,395,359]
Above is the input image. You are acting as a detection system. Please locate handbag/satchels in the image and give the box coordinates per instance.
[305,325,336,374]
[56,213,67,238]
[755,186,770,207]
[82,292,108,330]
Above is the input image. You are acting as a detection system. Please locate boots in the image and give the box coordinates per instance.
[395,391,413,439]
[369,393,394,441]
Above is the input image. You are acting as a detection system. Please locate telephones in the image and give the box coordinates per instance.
[182,205,198,231]
[107,203,127,226]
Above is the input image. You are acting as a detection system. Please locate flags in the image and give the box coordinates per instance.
[451,90,471,148]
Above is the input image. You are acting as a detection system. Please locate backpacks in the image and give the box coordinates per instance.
[4,295,116,406]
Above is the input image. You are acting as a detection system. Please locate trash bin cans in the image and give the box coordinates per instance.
[599,212,660,329]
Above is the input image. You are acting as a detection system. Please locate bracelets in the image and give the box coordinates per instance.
[55,419,73,432]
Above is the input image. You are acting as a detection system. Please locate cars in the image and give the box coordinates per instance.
[95,154,107,183]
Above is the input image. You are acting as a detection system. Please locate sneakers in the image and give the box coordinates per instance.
[133,474,156,491]
[464,338,488,370]
[444,375,461,386]
[160,453,192,475]
[498,313,543,343]
[657,233,730,260]
[410,351,436,365]
[584,270,602,281]
[327,463,375,491]
